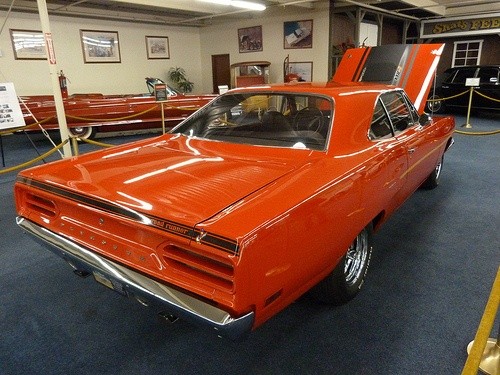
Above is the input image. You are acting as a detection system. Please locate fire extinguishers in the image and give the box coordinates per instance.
[58,70,68,99]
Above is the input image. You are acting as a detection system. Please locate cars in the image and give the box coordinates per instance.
[17,77,245,144]
[12,42,458,342]
[424,63,500,118]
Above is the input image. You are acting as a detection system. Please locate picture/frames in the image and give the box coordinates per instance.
[237,24,263,53]
[145,33,171,60]
[10,27,48,61]
[283,60,313,82]
[283,18,314,50]
[79,28,122,64]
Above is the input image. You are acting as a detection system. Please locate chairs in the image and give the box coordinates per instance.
[260,109,293,130]
